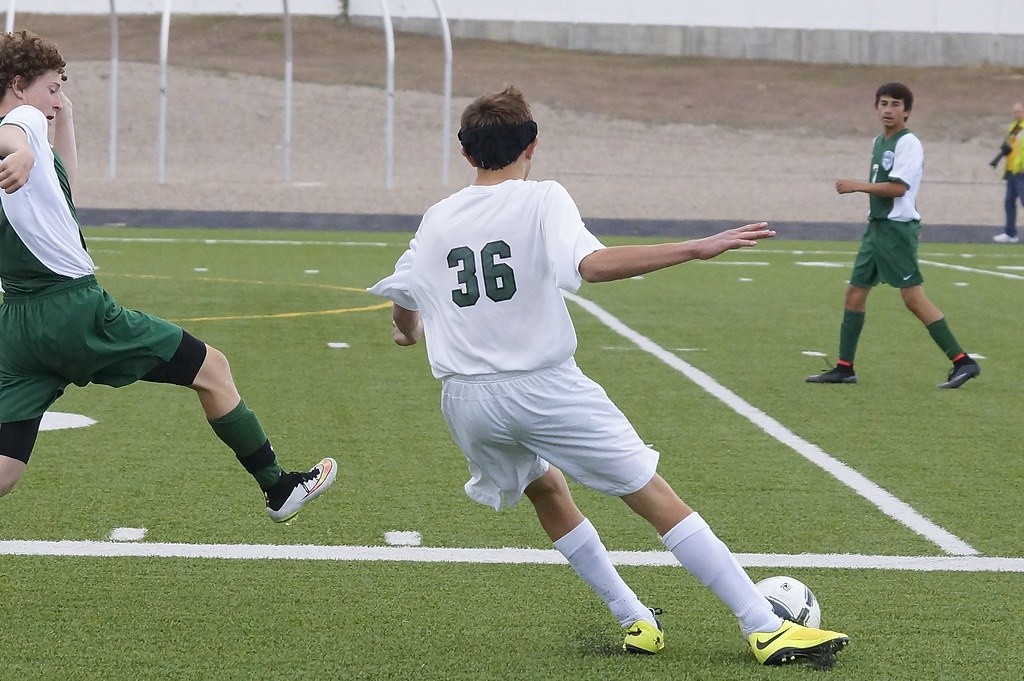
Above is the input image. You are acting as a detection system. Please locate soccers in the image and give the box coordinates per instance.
[738,575,822,651]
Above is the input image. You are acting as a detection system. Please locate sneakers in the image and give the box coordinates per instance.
[936,353,981,389]
[622,607,666,656]
[745,617,852,666]
[993,233,1019,244]
[805,363,857,383]
[265,455,337,523]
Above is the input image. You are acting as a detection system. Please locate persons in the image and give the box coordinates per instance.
[0,32,338,523]
[989,103,1024,244]
[804,84,980,390]
[365,82,850,666]
